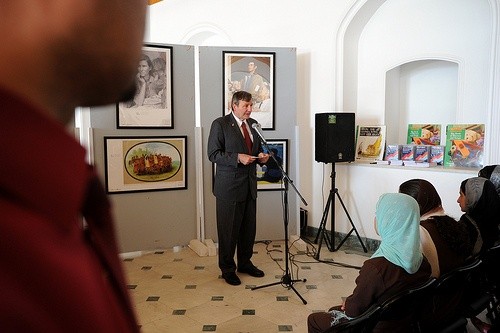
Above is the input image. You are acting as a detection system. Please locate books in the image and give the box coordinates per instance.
[385,144,444,164]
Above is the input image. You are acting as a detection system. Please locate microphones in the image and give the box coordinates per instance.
[252,123,267,143]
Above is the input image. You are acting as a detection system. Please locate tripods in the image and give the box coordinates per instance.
[249,141,309,305]
[311,163,368,260]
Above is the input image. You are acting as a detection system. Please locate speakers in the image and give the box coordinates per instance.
[315,113,355,162]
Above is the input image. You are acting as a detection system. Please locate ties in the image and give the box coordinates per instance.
[241,122,253,155]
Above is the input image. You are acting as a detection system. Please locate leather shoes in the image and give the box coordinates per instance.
[237,263,265,277]
[222,272,241,285]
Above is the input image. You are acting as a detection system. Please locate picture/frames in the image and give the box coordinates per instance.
[116,44,174,130]
[211,139,289,193]
[223,50,275,130]
[104,135,188,194]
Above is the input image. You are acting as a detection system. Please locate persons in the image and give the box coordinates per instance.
[398,179,473,281]
[478,165,500,197]
[0,0,163,333]
[457,177,500,256]
[207,91,269,285]
[308,192,433,333]
[257,149,282,183]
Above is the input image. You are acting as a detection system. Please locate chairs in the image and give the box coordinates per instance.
[322,239,500,333]
